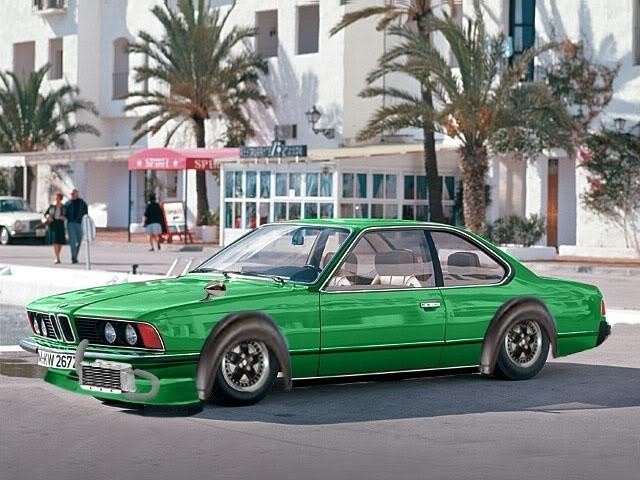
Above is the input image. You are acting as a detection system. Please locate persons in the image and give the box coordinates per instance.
[141,191,164,253]
[42,191,65,264]
[63,188,89,266]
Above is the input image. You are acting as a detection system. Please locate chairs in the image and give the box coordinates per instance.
[369,248,420,288]
[444,251,483,287]
[322,250,358,287]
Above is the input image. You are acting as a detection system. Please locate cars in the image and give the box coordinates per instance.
[15,214,614,424]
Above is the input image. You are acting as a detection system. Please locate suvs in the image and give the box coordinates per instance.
[0,194,47,247]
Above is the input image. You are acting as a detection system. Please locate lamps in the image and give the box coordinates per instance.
[304,103,332,136]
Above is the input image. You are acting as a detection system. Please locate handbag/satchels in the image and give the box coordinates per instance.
[41,214,55,225]
